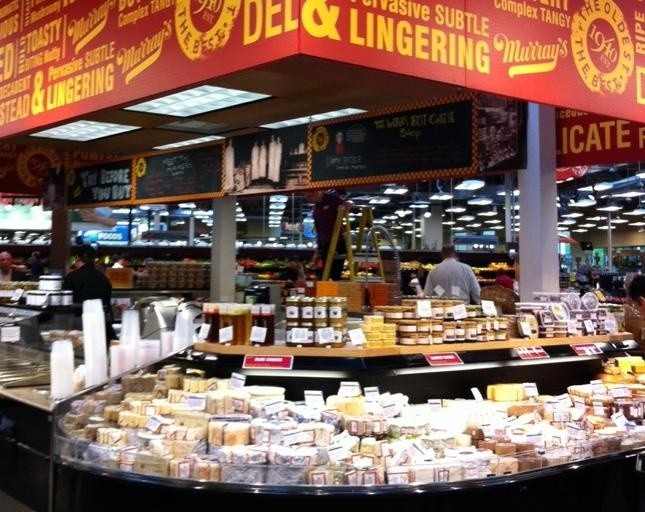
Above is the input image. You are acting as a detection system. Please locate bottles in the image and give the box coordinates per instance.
[203,303,275,346]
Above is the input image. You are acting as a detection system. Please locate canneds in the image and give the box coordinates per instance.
[374,299,509,344]
[286,296,348,347]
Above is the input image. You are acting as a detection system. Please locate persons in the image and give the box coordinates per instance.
[422,243,482,306]
[302,186,353,280]
[4,244,152,342]
[73,207,116,226]
[494,268,515,291]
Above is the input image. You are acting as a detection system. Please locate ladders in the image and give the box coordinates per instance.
[323,205,385,281]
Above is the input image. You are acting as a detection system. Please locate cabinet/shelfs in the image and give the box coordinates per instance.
[1,300,207,512]
[48,331,645,511]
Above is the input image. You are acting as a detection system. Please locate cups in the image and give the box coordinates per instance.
[81,298,107,388]
[111,309,192,375]
[49,340,75,399]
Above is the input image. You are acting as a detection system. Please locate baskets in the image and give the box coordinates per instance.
[481,285,519,314]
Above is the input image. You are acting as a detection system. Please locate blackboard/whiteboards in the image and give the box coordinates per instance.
[67,141,225,208]
[306,96,479,189]
